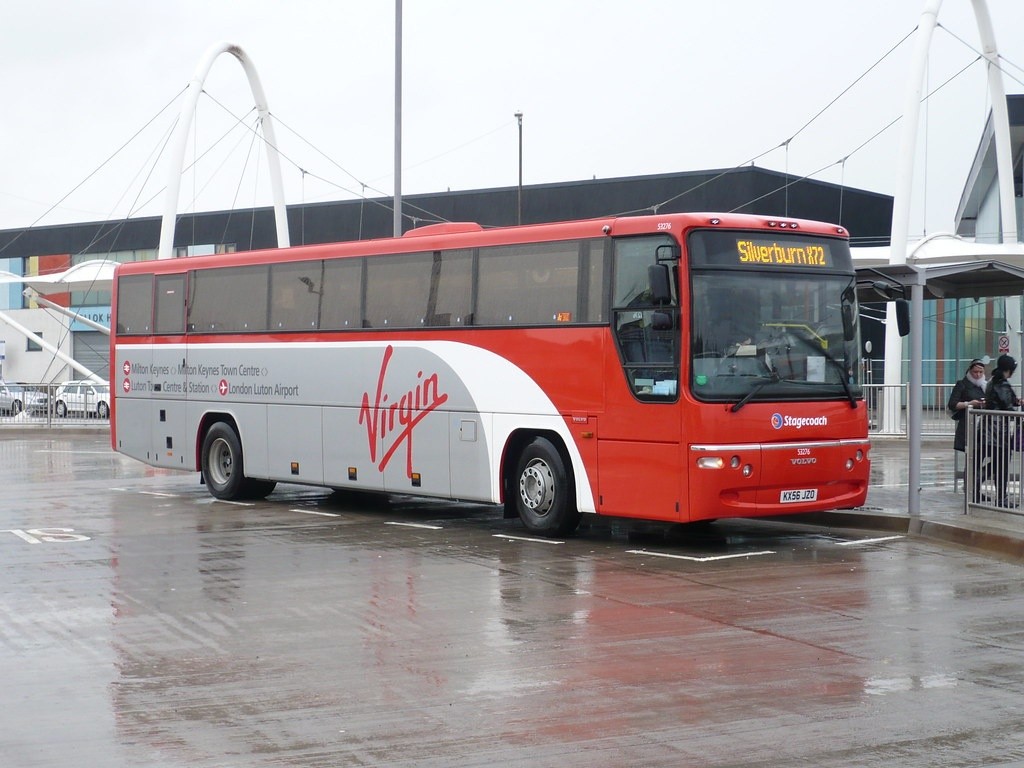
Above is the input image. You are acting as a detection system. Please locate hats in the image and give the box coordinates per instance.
[991,354,1018,376]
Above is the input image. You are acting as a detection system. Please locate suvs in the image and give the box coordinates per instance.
[56,380,110,417]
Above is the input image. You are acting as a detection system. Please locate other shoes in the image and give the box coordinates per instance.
[995,499,1020,508]
[973,492,992,503]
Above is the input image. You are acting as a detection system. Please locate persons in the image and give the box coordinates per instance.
[948,354,1024,508]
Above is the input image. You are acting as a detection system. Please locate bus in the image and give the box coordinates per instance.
[109,211,871,539]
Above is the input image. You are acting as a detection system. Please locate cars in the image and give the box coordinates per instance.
[0,382,54,415]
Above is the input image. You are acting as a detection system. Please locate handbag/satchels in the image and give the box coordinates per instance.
[1011,415,1023,451]
